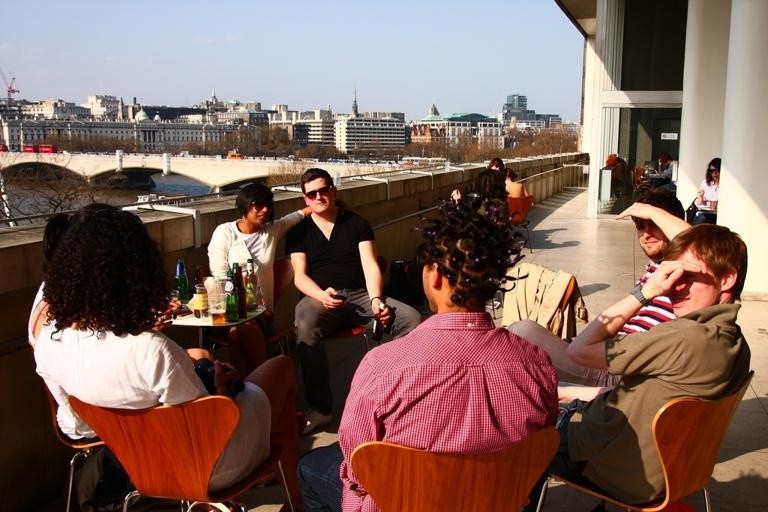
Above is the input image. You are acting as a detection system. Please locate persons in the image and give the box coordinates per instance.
[526,224,747,512]
[206,184,312,378]
[475,157,507,199]
[28,202,216,443]
[296,194,558,512]
[285,169,422,437]
[502,168,531,198]
[34,206,308,512]
[650,153,673,187]
[508,187,694,403]
[693,157,721,226]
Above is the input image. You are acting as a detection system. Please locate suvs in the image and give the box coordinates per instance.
[63,150,72,154]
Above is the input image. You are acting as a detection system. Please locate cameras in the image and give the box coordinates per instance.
[193,357,232,395]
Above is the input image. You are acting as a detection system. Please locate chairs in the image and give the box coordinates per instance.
[534,370,755,512]
[195,257,366,405]
[504,195,537,254]
[72,396,296,512]
[40,373,108,510]
[507,263,578,342]
[630,158,717,229]
[350,428,560,512]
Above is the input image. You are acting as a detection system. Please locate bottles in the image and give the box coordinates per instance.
[226,259,257,322]
[174,257,190,304]
[371,300,386,342]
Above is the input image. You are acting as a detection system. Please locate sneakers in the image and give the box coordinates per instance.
[301,407,334,433]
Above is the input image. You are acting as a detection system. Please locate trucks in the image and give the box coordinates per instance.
[0,144,9,152]
[226,152,244,160]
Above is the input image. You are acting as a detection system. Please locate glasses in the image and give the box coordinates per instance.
[306,186,333,199]
[710,169,720,173]
[252,200,273,210]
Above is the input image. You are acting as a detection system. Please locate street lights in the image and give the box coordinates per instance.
[202,121,212,154]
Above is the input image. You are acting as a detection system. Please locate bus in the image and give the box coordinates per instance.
[18,143,38,153]
[39,144,58,153]
[401,157,446,165]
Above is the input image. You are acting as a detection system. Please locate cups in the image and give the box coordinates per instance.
[193,277,227,324]
[710,200,717,209]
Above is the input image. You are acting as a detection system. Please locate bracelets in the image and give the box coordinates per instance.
[370,296,383,306]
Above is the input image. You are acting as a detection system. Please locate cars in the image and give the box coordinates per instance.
[306,159,317,162]
[97,151,110,155]
[80,151,89,155]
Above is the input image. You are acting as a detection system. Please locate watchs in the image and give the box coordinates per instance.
[631,285,651,306]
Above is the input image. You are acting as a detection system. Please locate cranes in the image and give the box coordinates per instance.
[0,66,20,99]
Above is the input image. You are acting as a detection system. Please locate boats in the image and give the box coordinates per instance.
[132,193,165,203]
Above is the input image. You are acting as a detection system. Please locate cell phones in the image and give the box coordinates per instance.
[332,292,347,300]
